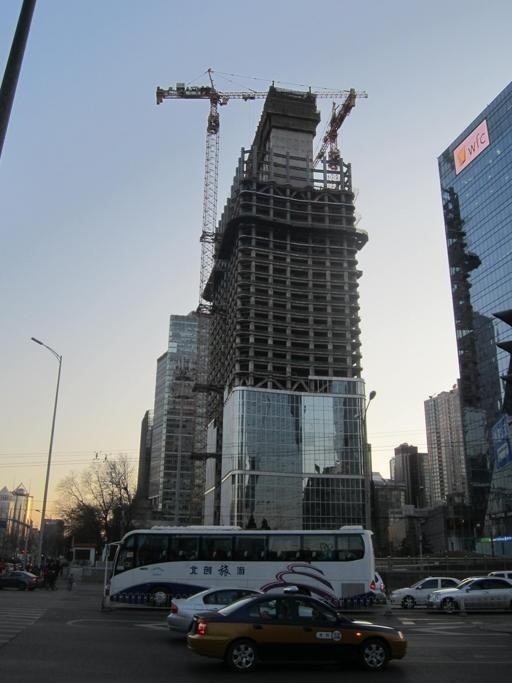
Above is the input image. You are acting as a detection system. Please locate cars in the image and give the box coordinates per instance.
[375,570,512,614]
[167,586,408,673]
[0,571,39,591]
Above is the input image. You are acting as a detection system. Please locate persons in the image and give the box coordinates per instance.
[17,553,64,591]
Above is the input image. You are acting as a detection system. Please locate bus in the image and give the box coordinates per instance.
[100,524,376,609]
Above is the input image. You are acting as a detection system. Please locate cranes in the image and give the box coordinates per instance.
[155,67,370,315]
[312,88,357,188]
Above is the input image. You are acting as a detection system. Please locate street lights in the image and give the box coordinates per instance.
[31,337,63,569]
[365,390,378,411]
